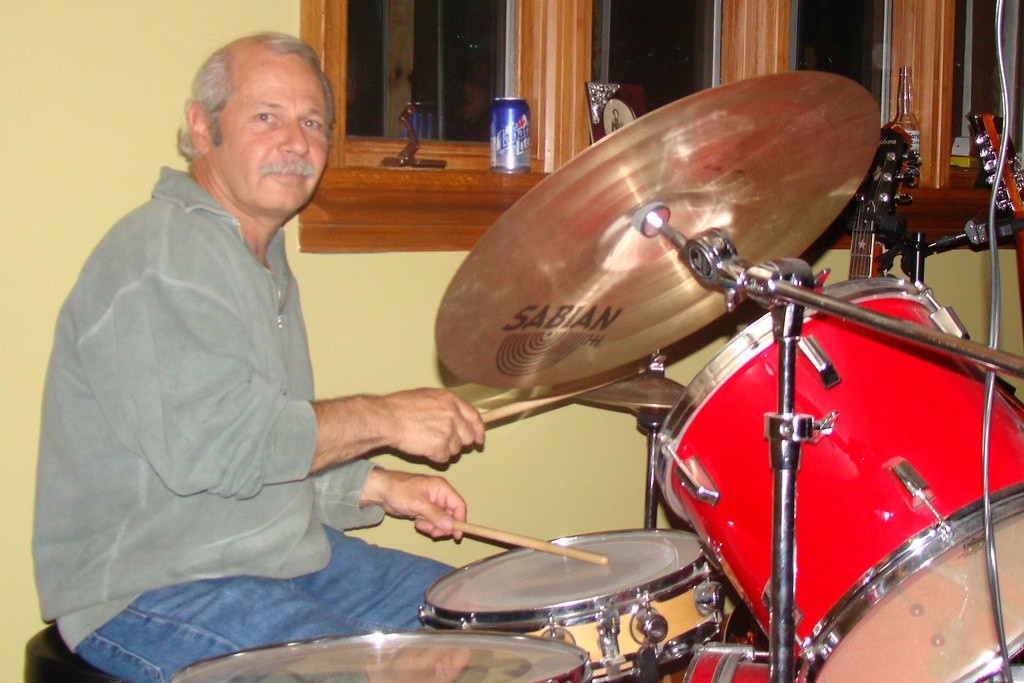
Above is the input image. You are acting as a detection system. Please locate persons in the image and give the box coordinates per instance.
[611,109,625,132]
[32,30,483,683]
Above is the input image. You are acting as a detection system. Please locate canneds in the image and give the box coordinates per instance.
[492,96,532,172]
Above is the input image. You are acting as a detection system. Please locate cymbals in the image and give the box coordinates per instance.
[575,369,685,415]
[431,68,883,391]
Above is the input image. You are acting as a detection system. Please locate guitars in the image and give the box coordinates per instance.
[847,120,923,282]
[964,110,1023,302]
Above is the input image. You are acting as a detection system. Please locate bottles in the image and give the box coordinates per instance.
[894,67,919,187]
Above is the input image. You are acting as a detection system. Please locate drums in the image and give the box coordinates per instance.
[169,626,592,683]
[681,638,771,682]
[418,528,727,683]
[649,273,1024,683]
